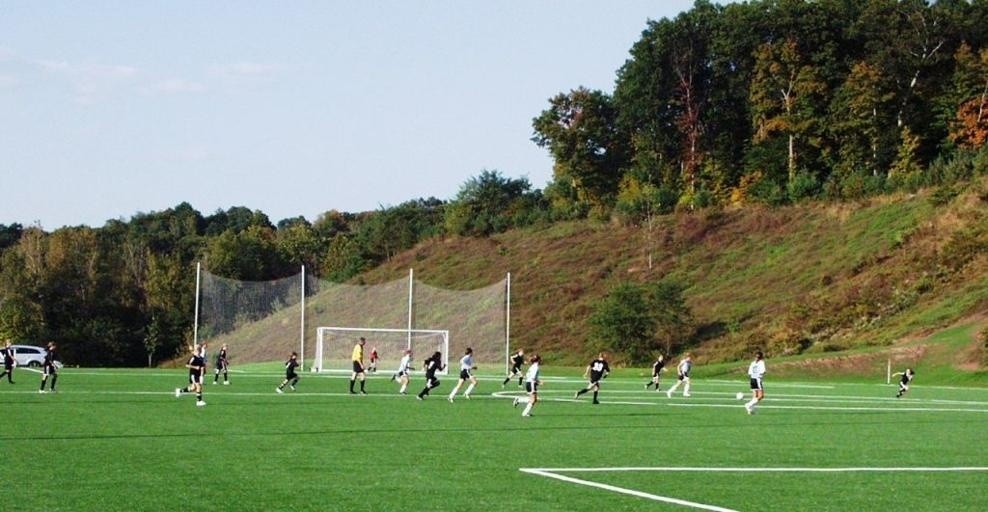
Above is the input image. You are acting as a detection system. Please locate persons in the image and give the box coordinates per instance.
[643,354,670,393]
[448,348,477,403]
[0,338,18,386]
[513,355,542,416]
[743,351,768,415]
[502,350,525,388]
[213,345,230,385]
[890,367,916,398]
[392,350,416,395]
[664,351,697,400]
[37,340,60,394]
[368,348,378,372]
[277,352,301,393]
[349,337,366,393]
[573,351,611,405]
[416,352,446,400]
[176,342,209,406]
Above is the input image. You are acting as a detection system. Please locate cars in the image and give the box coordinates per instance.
[52,360,63,368]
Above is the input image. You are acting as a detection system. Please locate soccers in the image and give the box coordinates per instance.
[737,391,745,401]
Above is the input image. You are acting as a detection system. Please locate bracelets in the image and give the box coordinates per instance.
[602,374,608,379]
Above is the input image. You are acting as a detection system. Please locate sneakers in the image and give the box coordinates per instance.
[745,403,755,415]
[683,393,690,396]
[224,381,229,384]
[276,387,284,392]
[667,392,671,398]
[522,413,534,417]
[513,397,518,406]
[176,388,181,398]
[196,400,206,406]
[399,387,471,403]
[213,382,217,384]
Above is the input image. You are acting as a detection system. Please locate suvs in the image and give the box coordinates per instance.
[0,344,48,367]
